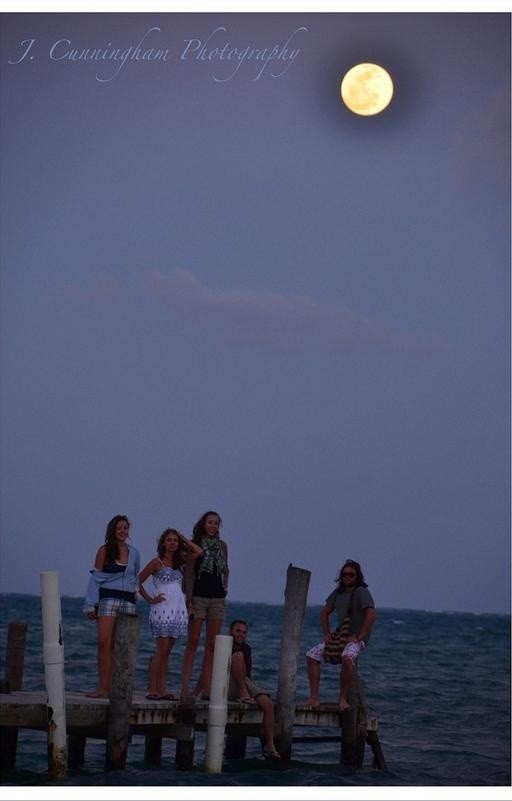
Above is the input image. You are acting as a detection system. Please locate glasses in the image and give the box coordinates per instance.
[342,572,355,577]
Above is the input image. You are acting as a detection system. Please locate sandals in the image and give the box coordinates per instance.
[162,692,179,702]
[263,749,280,760]
[84,689,108,699]
[200,693,211,702]
[145,693,162,701]
[237,696,257,706]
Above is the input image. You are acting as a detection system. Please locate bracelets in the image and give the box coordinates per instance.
[355,635,360,643]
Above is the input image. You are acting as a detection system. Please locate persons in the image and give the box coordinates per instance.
[180,509,227,699]
[224,618,284,763]
[296,555,376,712]
[79,510,142,700]
[133,526,205,702]
